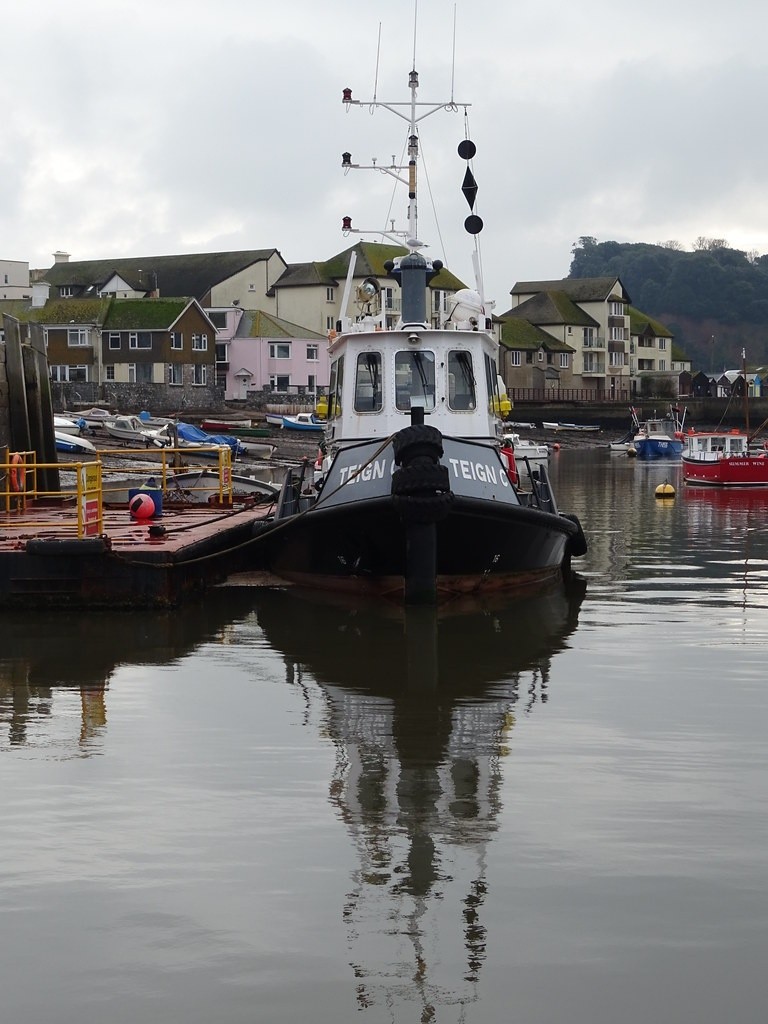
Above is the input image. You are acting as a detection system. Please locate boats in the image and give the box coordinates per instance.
[53,407,279,459]
[502,434,548,459]
[264,413,283,425]
[0,444,281,607]
[282,413,328,430]
[502,421,535,429]
[629,403,687,461]
[542,422,601,431]
[611,426,634,450]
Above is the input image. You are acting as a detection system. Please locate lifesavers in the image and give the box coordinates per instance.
[10,454,25,492]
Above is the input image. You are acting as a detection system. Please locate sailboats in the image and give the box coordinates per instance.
[681,347,768,486]
[242,0,589,602]
